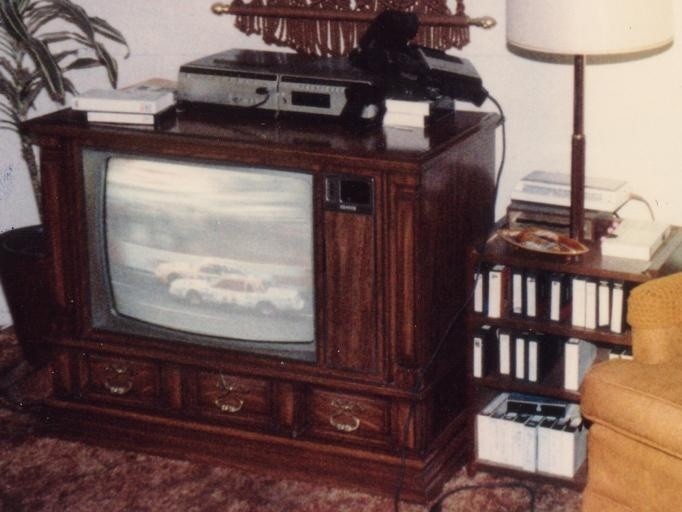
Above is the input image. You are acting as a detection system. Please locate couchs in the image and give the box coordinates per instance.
[577,273,682,511]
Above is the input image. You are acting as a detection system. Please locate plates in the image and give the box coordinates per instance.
[496,227,585,259]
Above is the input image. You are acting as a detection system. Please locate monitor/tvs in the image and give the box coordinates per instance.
[83,149,318,362]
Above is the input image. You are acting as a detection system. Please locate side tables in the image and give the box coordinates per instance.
[455,214,682,476]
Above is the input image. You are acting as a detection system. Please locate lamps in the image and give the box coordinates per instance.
[507,1,674,263]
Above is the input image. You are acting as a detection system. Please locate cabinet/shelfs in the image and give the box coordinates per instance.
[18,78,504,505]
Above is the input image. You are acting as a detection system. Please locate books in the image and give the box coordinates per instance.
[71,78,177,125]
[381,95,456,129]
[473,265,633,391]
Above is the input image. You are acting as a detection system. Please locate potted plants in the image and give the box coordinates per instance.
[0,0,131,365]
